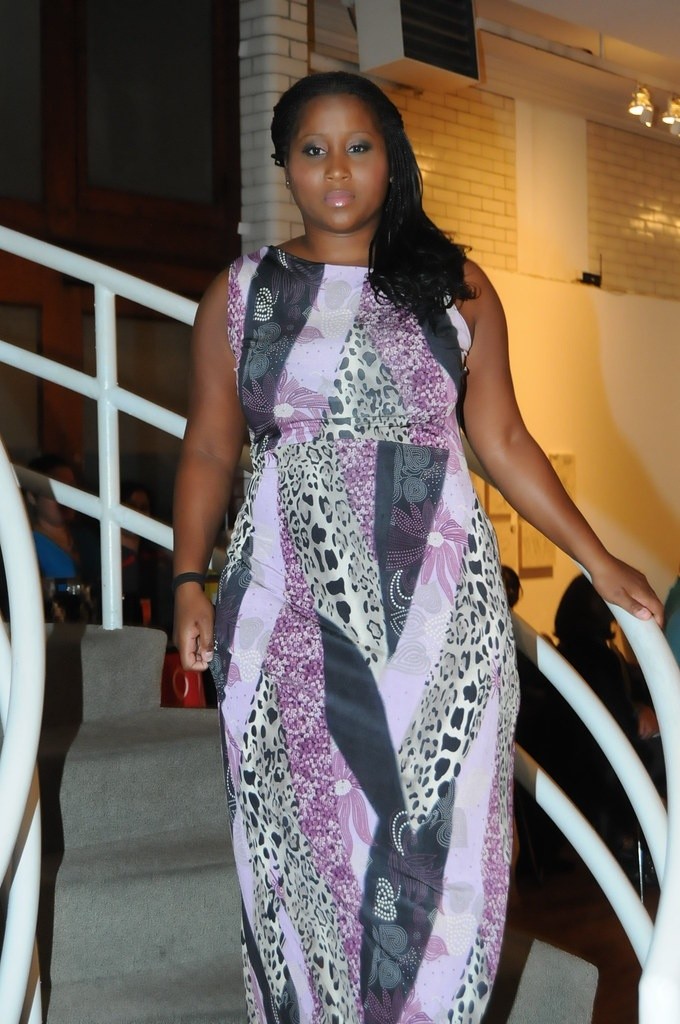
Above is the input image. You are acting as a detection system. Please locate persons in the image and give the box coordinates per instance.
[0,456,158,629]
[170,72,664,1024]
[513,573,667,885]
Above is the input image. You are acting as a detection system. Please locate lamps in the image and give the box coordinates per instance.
[662,96,680,124]
[627,86,654,116]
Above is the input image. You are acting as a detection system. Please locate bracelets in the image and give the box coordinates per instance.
[173,572,206,590]
